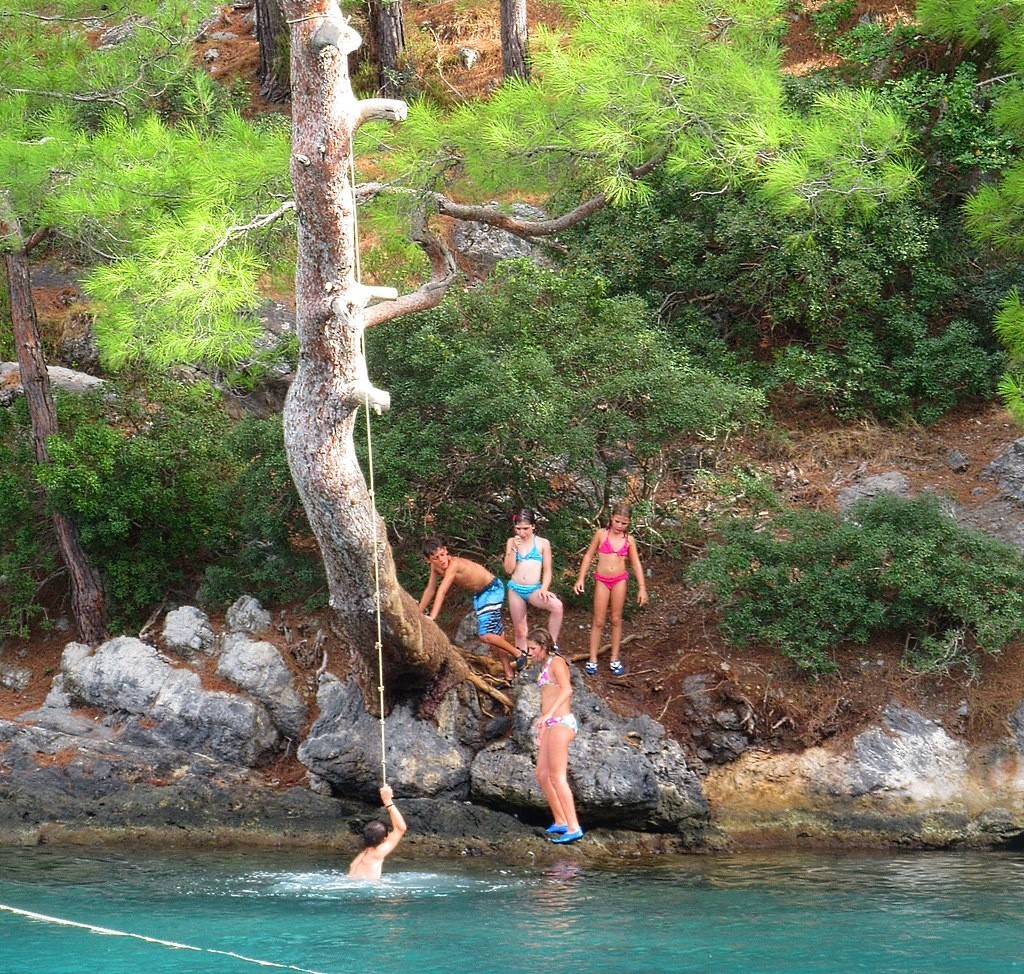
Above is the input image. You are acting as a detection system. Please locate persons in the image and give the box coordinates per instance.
[415,538,528,690]
[346,782,407,884]
[503,507,563,673]
[574,502,649,675]
[526,628,584,844]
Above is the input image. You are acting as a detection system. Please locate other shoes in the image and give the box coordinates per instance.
[610,660,624,675]
[553,642,560,654]
[545,825,568,833]
[515,647,527,672]
[495,678,513,691]
[586,662,598,674]
[551,828,584,843]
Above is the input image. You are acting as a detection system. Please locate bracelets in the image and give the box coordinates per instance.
[510,547,518,554]
[386,804,395,809]
